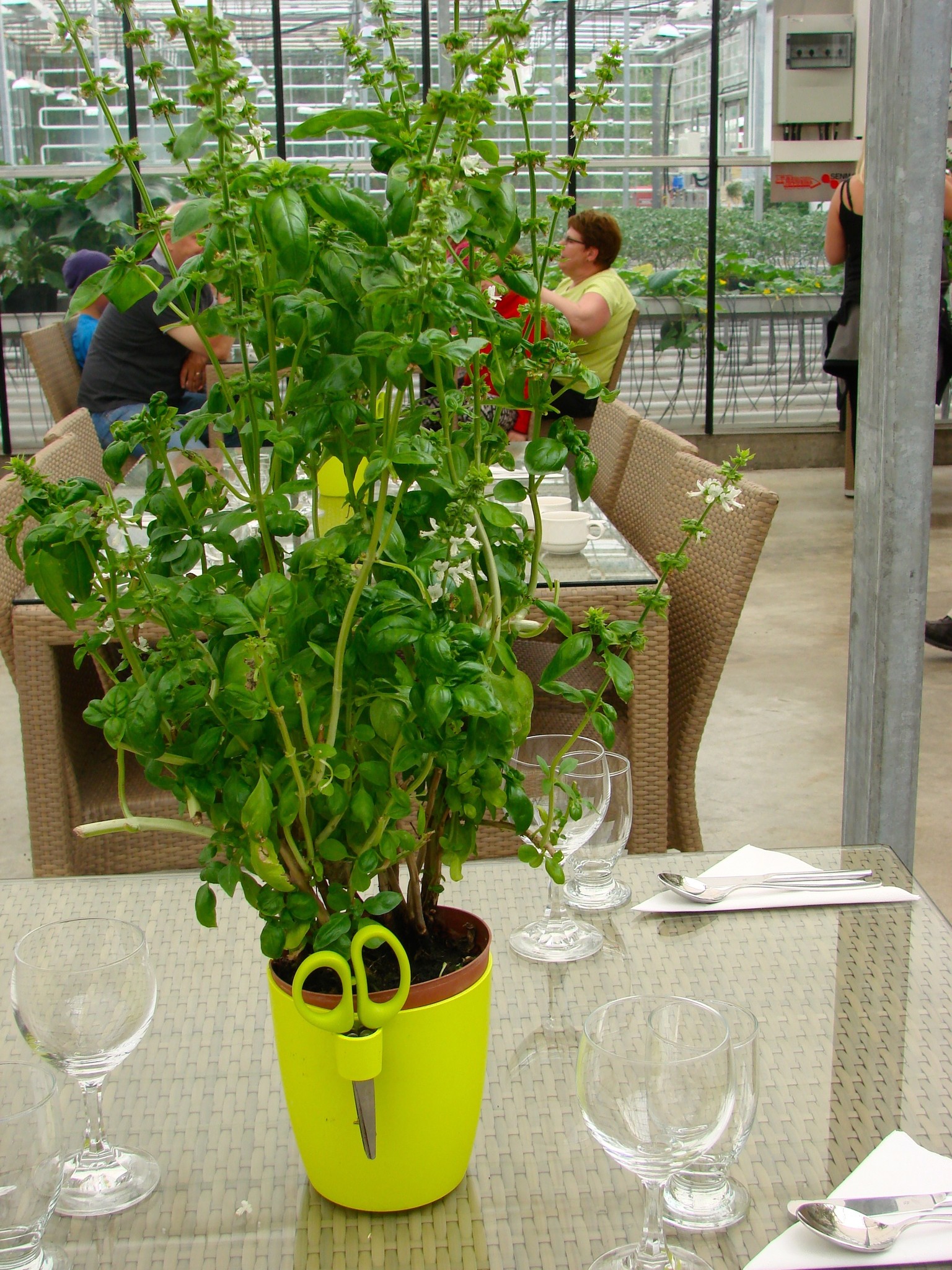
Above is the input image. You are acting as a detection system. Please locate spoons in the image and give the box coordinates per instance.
[795,1203,952,1253]
[657,873,882,904]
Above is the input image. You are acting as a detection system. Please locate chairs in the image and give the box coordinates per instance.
[0,315,778,851]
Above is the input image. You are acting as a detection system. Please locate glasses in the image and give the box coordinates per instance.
[563,232,585,245]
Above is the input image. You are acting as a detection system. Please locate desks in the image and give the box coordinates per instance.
[14,441,670,876]
[0,845,952,1270]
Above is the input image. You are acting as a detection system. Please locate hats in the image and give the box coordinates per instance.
[62,249,113,295]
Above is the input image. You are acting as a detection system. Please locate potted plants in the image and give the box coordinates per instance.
[0,0,760,1214]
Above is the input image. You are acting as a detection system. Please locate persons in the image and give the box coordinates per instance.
[924,609,952,651]
[75,201,235,458]
[408,174,637,443]
[823,119,952,483]
[62,249,113,370]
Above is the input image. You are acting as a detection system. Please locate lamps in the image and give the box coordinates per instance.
[0,0,713,116]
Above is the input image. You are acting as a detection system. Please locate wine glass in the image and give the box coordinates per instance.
[498,733,611,963]
[575,993,715,1270]
[8,917,160,1218]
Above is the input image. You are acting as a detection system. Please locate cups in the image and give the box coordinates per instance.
[554,750,633,910]
[520,494,605,555]
[1,1061,68,1269]
[218,449,272,510]
[650,998,762,1233]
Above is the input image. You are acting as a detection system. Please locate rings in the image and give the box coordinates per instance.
[196,372,200,375]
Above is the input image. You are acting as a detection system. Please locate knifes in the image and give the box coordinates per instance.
[786,1191,952,1219]
[689,868,872,889]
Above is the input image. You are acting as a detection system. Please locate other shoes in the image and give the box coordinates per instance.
[925,616,952,651]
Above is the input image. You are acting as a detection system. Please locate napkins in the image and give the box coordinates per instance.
[744,1130,952,1270]
[631,844,921,913]
